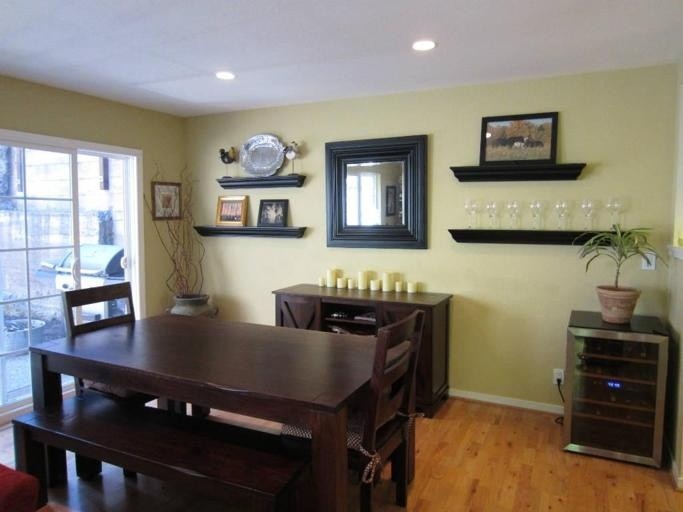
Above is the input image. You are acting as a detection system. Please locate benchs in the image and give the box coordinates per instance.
[11,389,314,512]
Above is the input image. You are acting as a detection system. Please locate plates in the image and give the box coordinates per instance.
[237,134,284,176]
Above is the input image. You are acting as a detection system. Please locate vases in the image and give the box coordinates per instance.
[4,317,46,358]
[164,292,218,320]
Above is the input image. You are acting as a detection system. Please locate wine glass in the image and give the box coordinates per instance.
[462,197,621,231]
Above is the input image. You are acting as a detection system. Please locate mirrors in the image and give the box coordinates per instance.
[324,134,426,250]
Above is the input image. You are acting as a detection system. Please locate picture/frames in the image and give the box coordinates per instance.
[257,198,289,227]
[215,195,248,226]
[479,112,557,164]
[151,181,182,221]
[385,186,396,216]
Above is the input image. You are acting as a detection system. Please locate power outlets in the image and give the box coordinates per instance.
[553,368,564,385]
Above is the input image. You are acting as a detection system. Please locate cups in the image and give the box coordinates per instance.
[316,267,418,294]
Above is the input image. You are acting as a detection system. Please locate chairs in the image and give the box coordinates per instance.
[279,309,425,510]
[0,462,37,512]
[61,280,159,477]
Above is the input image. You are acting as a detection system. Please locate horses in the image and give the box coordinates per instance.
[492,136,544,150]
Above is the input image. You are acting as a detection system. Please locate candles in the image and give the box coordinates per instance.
[316,267,419,295]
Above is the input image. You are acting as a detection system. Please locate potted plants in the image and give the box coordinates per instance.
[571,222,669,323]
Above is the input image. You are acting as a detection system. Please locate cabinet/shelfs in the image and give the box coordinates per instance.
[444,161,636,245]
[191,175,308,239]
[271,282,454,417]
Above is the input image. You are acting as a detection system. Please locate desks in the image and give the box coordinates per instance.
[26,312,417,510]
